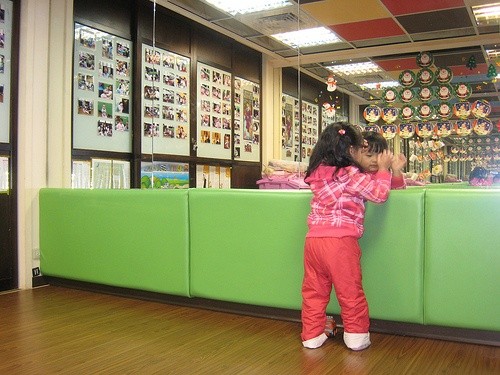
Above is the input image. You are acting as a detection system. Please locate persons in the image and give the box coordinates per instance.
[71,20,335,161]
[300,121,394,349]
[360,131,408,189]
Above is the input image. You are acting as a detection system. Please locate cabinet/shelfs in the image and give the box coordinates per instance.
[39,185,499,347]
